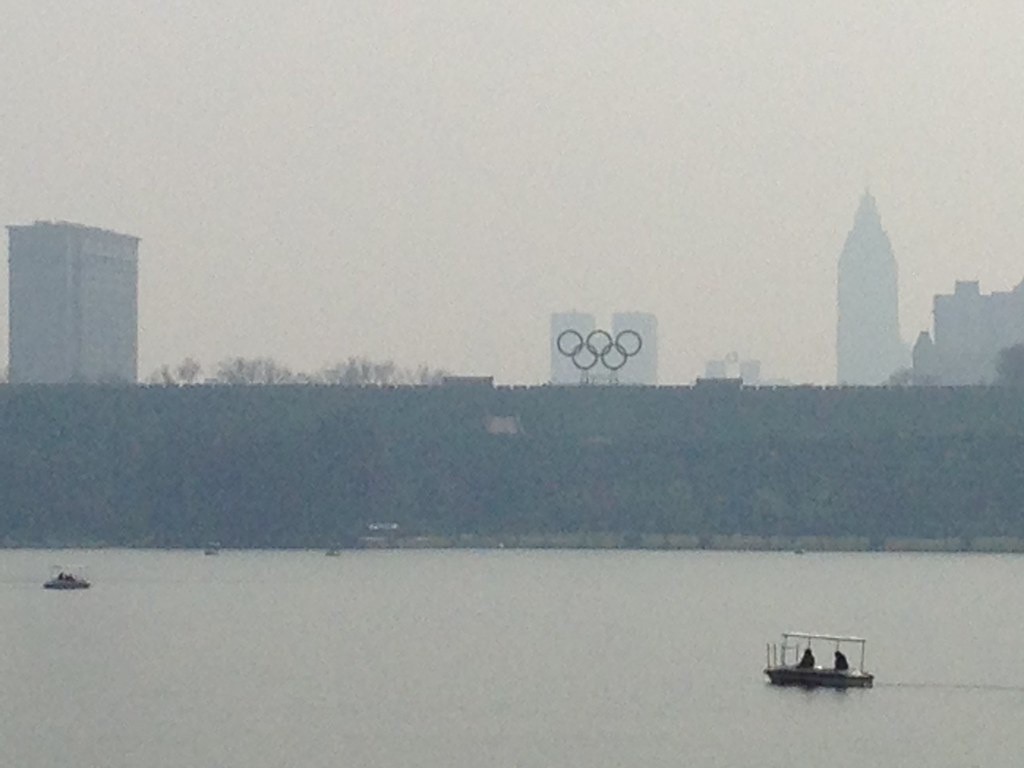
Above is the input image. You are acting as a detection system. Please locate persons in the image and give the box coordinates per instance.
[57,571,74,581]
[797,649,814,668]
[833,650,849,670]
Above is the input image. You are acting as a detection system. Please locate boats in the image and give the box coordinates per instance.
[763,632,875,688]
[43,577,91,589]
